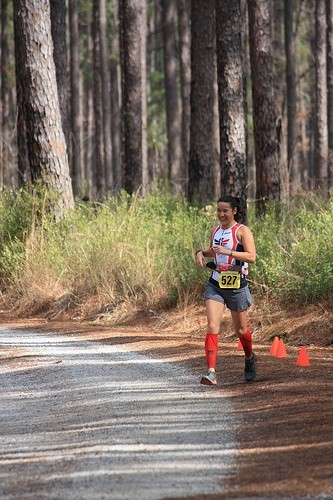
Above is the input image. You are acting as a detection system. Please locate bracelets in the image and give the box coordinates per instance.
[195,250,206,256]
[229,250,234,257]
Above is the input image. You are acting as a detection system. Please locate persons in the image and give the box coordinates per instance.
[195,196,257,385]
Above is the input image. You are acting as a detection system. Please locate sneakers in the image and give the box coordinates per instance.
[244,352,256,381]
[200,371,217,385]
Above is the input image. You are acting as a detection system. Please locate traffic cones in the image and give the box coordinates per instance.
[270,336,282,354]
[272,338,289,358]
[295,346,310,367]
[237,340,243,351]
